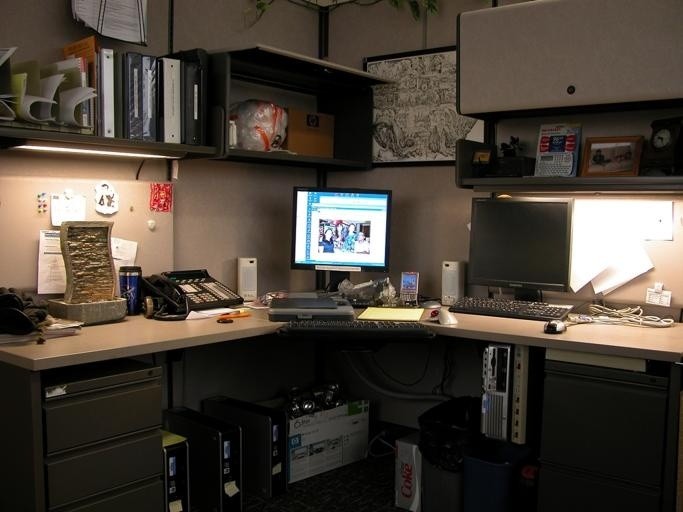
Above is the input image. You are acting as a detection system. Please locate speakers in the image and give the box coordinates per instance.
[440,261,465,307]
[237,257,258,303]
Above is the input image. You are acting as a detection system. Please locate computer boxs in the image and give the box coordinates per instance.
[201,394,286,507]
[161,429,191,512]
[163,404,242,510]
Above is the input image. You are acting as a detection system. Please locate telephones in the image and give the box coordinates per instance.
[142,269,244,320]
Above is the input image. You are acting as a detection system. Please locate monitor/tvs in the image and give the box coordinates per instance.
[465,196,573,302]
[290,185,393,292]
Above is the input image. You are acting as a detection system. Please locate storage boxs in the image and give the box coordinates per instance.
[255,396,371,484]
[282,107,336,159]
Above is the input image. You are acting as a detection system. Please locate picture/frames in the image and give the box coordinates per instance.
[362,45,494,168]
[581,133,644,178]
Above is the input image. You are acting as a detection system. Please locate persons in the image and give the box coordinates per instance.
[318,223,369,253]
[97,184,114,208]
[593,149,605,164]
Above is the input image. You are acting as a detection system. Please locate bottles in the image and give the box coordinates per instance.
[286,387,302,418]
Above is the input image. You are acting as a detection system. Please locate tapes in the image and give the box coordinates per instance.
[144,296,153,318]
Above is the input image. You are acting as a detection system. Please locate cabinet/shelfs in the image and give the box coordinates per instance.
[43,366,165,512]
[537,358,680,512]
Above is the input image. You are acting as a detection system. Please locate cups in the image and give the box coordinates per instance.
[118,265,141,314]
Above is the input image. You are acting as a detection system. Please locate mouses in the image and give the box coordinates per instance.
[545,320,569,334]
[436,310,457,328]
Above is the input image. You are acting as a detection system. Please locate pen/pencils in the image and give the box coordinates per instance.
[217,313,252,320]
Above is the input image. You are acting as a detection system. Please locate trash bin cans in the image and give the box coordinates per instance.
[418,394,483,511]
[461,440,528,512]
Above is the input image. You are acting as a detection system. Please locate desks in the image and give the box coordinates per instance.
[0,291,683,511]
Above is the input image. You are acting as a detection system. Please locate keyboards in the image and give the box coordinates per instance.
[449,297,574,324]
[279,320,434,335]
